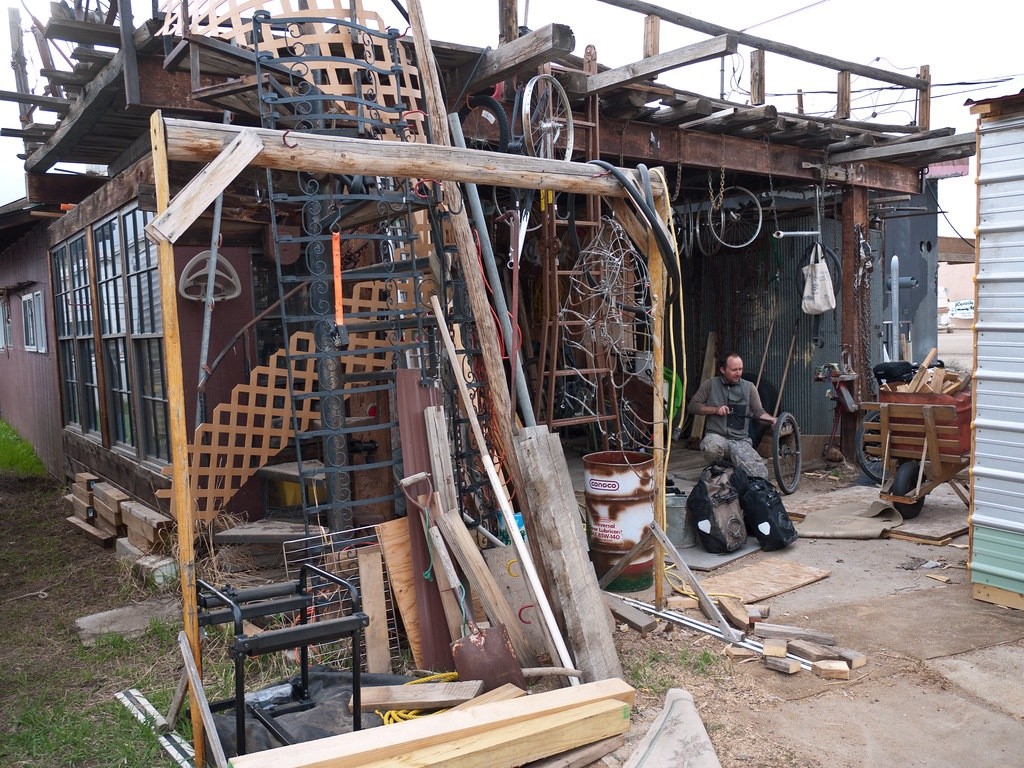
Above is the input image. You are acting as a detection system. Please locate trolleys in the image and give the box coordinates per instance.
[860,381,971,519]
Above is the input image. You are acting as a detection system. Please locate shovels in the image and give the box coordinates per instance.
[396,471,530,696]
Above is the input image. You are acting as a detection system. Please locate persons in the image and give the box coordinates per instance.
[687,351,778,481]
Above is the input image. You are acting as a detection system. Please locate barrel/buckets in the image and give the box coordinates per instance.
[582,448,656,593]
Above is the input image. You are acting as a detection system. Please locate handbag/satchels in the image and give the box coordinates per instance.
[802,244,836,315]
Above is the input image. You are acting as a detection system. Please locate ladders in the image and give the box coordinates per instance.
[534,44,624,455]
